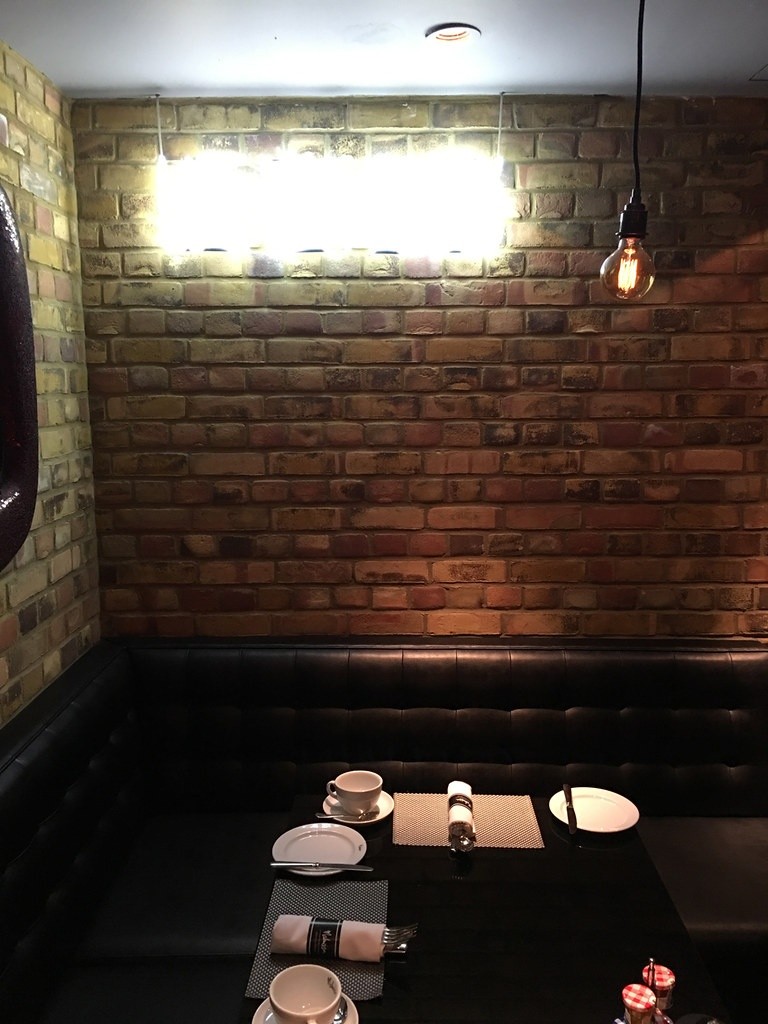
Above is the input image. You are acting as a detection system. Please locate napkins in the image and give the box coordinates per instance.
[269,914,386,964]
[448,780,476,838]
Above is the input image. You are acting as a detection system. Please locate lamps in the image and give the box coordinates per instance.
[599,0,655,301]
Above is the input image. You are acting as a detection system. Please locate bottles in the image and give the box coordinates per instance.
[623,984,656,1024]
[642,964,675,1012]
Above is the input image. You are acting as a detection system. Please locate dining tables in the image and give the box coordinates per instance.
[1,769,717,1024]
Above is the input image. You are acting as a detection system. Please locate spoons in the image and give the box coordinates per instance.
[316,811,379,821]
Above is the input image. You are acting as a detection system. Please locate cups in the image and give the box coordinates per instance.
[268,964,342,1024]
[327,770,383,815]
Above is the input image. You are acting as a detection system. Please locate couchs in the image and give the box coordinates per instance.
[0,635,767,1024]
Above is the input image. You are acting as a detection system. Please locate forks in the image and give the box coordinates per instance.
[382,923,418,943]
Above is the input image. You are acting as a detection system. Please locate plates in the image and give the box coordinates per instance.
[252,991,359,1024]
[323,790,394,824]
[549,787,639,832]
[272,823,367,875]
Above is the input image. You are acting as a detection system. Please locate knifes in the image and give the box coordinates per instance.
[563,783,577,834]
[270,861,374,871]
